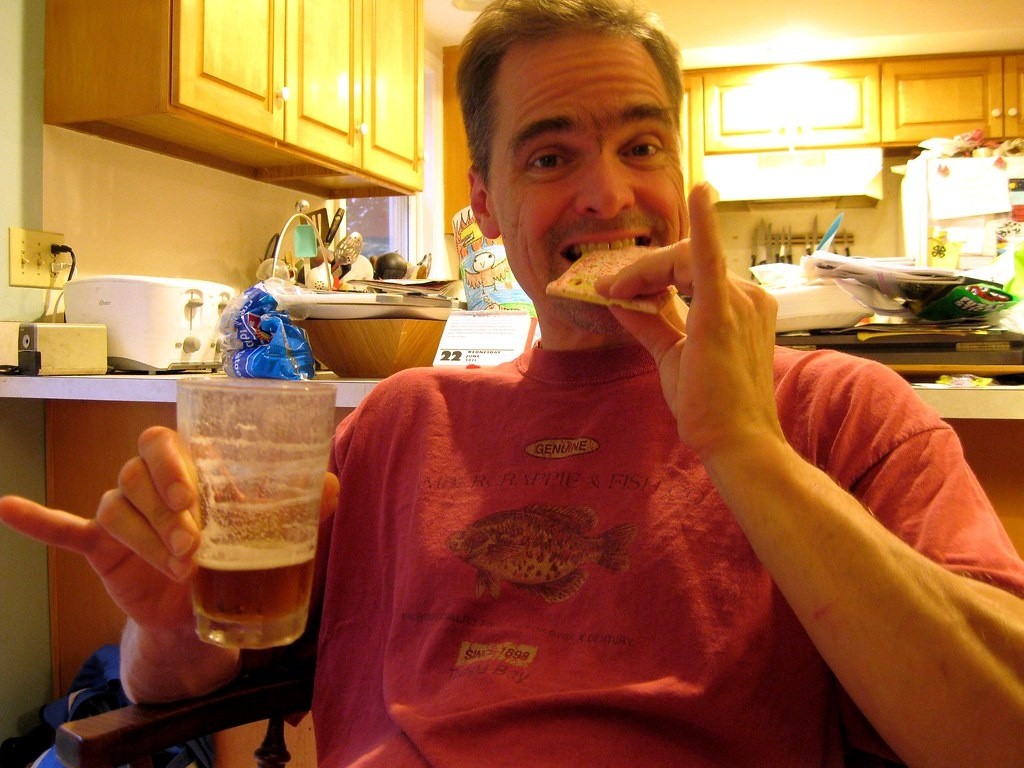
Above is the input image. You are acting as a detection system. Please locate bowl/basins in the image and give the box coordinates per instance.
[292,319,447,381]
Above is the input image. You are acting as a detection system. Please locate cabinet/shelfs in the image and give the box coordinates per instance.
[43,0,425,201]
[703,57,882,154]
[674,68,703,203]
[881,48,1024,153]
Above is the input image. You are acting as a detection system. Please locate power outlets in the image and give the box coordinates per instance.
[8,226,73,290]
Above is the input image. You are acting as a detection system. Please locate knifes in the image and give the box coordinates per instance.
[803,216,818,254]
[842,229,851,257]
[776,224,793,264]
[832,229,838,257]
[751,221,771,263]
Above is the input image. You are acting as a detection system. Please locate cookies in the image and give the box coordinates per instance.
[546,244,678,313]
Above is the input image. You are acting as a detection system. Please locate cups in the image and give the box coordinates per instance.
[177,378,338,650]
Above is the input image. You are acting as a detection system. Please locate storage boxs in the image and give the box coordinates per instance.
[290,319,446,378]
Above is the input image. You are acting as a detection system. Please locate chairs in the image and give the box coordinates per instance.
[51,662,312,768]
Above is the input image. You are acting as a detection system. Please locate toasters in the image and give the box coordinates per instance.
[67,274,235,372]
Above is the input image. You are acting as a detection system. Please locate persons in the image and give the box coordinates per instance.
[0,1,1024,768]
